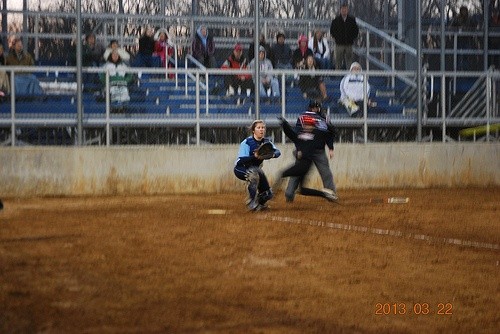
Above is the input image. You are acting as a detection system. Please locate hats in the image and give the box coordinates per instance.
[234,45,241,52]
[309,101,321,108]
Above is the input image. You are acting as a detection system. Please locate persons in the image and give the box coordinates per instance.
[299,56,326,101]
[221,44,253,98]
[83,33,95,50]
[272,34,292,62]
[248,34,270,62]
[0,41,42,95]
[135,26,174,68]
[233,120,281,212]
[293,35,313,66]
[339,62,370,115]
[312,30,331,60]
[99,39,136,103]
[330,5,359,70]
[277,104,340,203]
[193,24,218,69]
[248,45,280,97]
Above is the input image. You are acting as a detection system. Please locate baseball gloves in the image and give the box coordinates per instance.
[257,141,274,159]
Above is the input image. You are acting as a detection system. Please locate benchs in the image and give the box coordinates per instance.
[0,57,405,118]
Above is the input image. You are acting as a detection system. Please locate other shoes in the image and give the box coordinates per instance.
[274,98,281,103]
[324,96,329,101]
[265,99,271,105]
[286,194,293,202]
